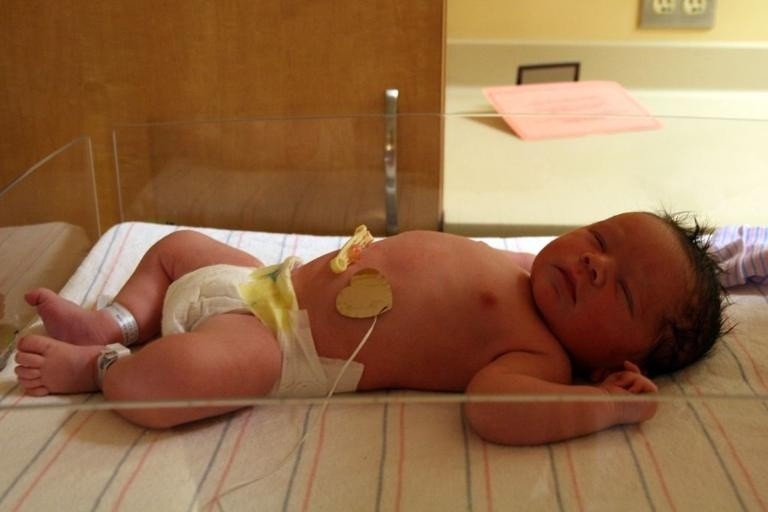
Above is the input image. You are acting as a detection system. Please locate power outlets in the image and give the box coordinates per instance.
[636,0,719,33]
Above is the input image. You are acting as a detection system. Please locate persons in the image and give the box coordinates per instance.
[13,208,741,446]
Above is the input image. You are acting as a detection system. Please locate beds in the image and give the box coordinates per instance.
[1,97,768,510]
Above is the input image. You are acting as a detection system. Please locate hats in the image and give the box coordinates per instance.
[693,225,768,289]
